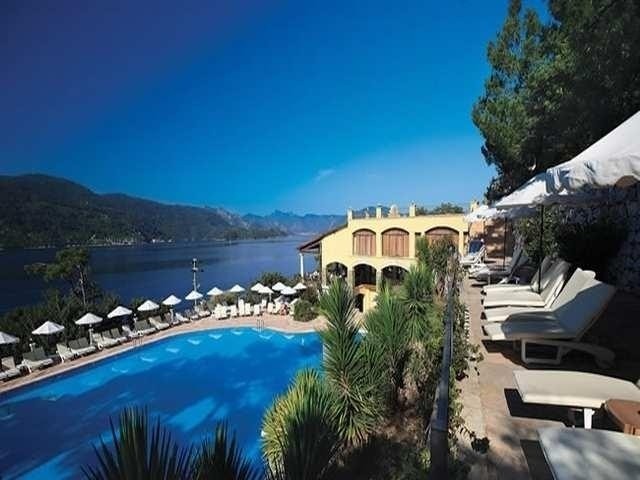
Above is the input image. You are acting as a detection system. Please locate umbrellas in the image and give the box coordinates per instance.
[477,206,537,271]
[546,110,640,198]
[496,172,609,295]
[463,205,497,245]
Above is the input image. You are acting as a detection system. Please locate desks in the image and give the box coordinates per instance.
[603,398,640,435]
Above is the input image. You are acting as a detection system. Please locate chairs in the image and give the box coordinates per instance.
[482,255,596,322]
[537,427,639,479]
[465,240,531,285]
[0,296,300,385]
[512,369,640,430]
[485,280,615,366]
